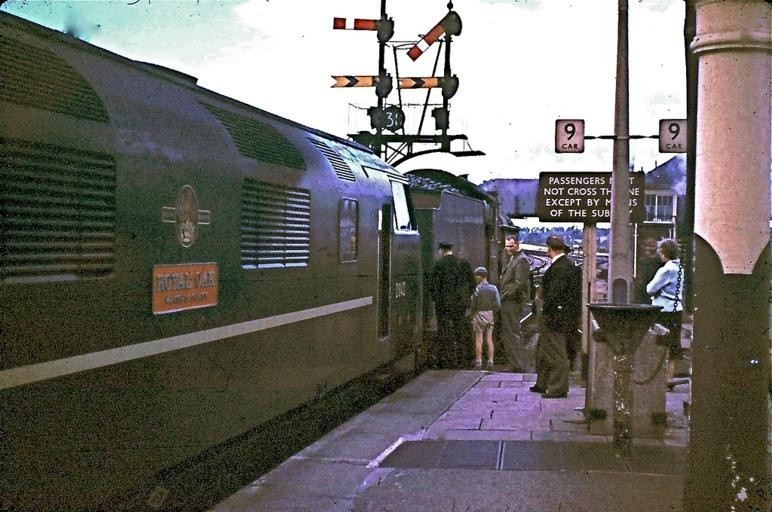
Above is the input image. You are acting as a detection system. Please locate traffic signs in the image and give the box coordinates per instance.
[539,174,644,224]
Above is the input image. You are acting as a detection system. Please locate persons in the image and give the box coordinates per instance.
[500,231,531,375]
[528,233,576,399]
[645,237,685,391]
[467,265,501,374]
[428,240,476,370]
[563,244,583,370]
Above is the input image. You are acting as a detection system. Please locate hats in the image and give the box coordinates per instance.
[437,240,454,248]
[546,234,571,254]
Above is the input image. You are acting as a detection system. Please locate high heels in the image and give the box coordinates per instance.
[668,382,675,390]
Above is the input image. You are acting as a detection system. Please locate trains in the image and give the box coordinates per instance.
[1,8,524,510]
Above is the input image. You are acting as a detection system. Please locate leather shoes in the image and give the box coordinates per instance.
[530,384,567,398]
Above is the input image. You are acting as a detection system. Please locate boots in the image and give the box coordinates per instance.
[486,360,494,369]
[475,360,482,368]
